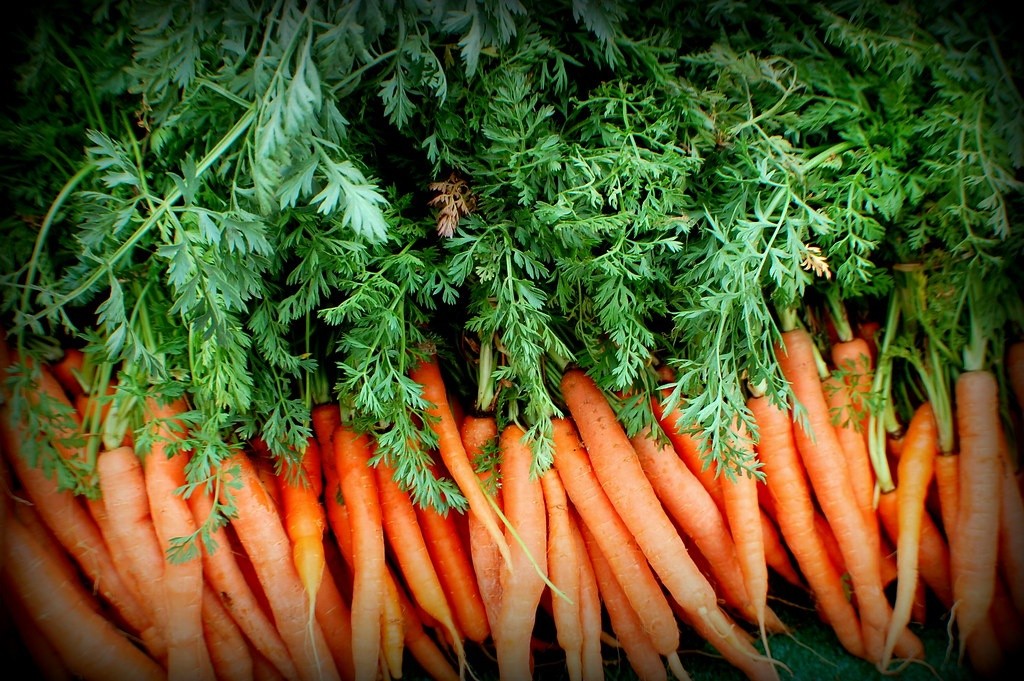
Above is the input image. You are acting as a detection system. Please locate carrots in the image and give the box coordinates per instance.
[0,301,1024,681]
